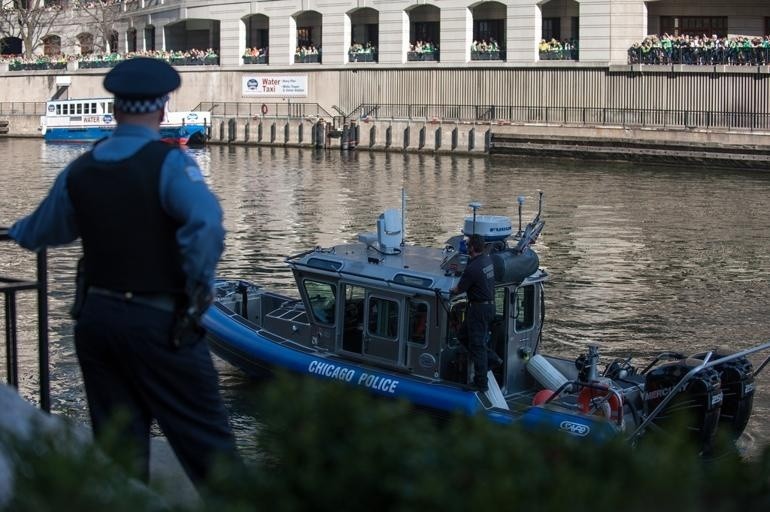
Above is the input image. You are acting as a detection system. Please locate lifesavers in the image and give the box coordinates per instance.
[577,383,623,427]
[262,104,268,115]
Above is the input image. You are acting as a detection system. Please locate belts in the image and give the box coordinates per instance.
[88,284,181,312]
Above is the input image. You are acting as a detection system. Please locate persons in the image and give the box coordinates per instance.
[243,46,270,63]
[5,54,268,512]
[0,46,220,73]
[407,39,440,61]
[348,41,379,64]
[446,231,504,395]
[538,35,579,62]
[627,29,770,67]
[293,42,323,63]
[470,37,505,59]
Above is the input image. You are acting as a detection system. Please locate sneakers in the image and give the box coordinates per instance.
[464,355,503,391]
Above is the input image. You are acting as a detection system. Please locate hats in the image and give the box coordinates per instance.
[102,57,181,112]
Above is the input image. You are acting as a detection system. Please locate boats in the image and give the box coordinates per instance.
[41,97,212,144]
[199,187,770,456]
